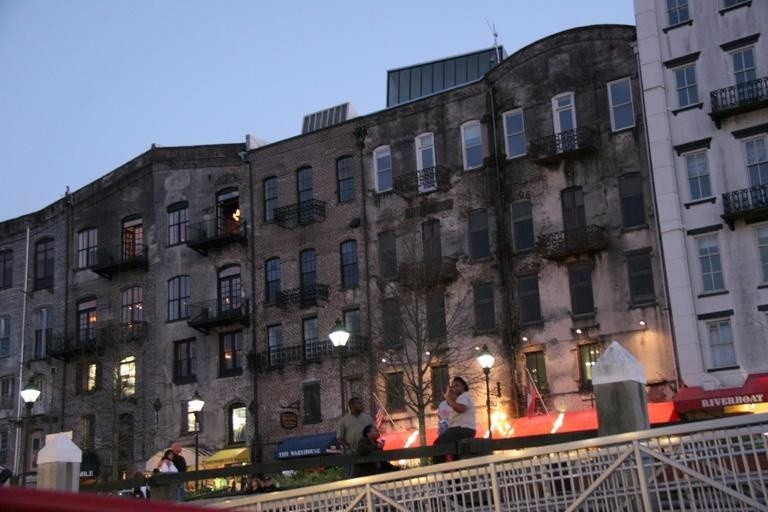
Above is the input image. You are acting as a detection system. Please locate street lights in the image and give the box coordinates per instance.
[476,343,495,438]
[19,383,41,486]
[188,390,205,493]
[327,316,350,416]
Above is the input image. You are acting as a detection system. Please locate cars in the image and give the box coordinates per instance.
[108,489,134,496]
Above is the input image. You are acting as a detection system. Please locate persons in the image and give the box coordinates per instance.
[427,375,476,462]
[336,396,374,478]
[248,477,278,494]
[352,424,400,477]
[133,441,188,501]
